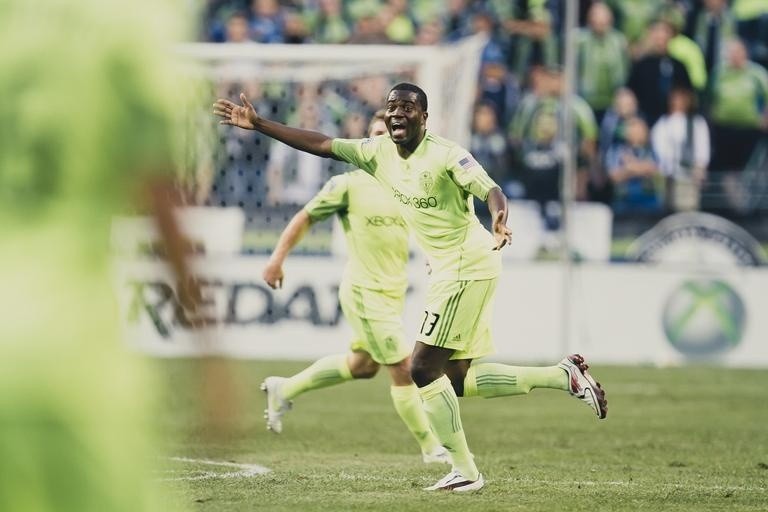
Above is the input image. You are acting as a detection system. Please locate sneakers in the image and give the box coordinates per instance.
[424,469,484,493]
[421,445,475,465]
[265,375,293,434]
[556,352,609,420]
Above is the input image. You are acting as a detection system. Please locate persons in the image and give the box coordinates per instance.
[1,1,244,511]
[259,108,473,467]
[216,0,767,225]
[212,81,607,493]
